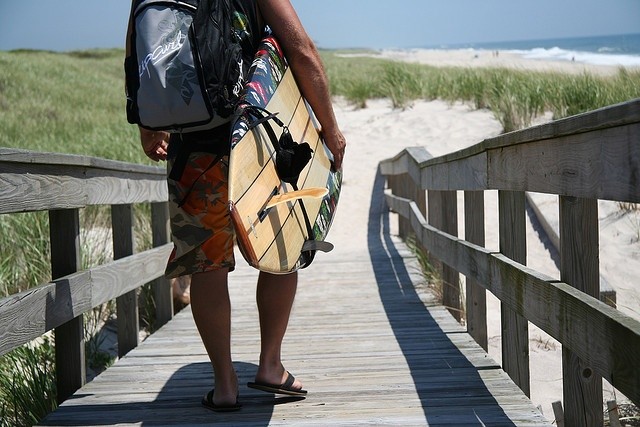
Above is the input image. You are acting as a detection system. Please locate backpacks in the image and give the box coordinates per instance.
[123,0,256,134]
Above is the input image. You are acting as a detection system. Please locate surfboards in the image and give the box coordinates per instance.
[227,29,343,275]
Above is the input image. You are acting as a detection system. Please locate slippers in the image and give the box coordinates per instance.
[202,387,240,411]
[248,370,307,395]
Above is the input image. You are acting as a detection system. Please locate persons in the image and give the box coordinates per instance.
[124,0,347,414]
